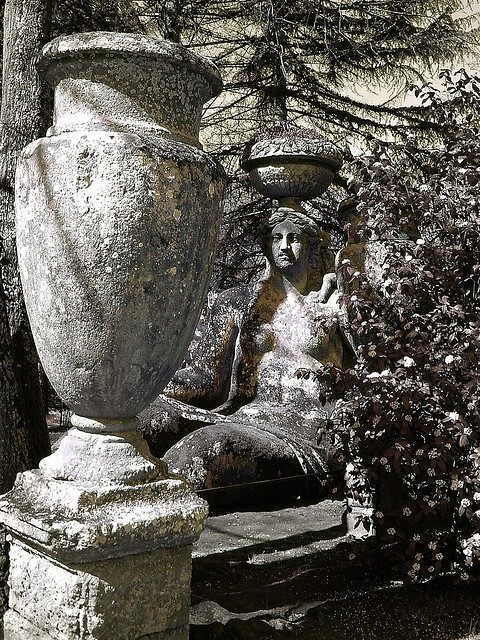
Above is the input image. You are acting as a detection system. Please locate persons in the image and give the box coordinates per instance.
[139,208,355,493]
